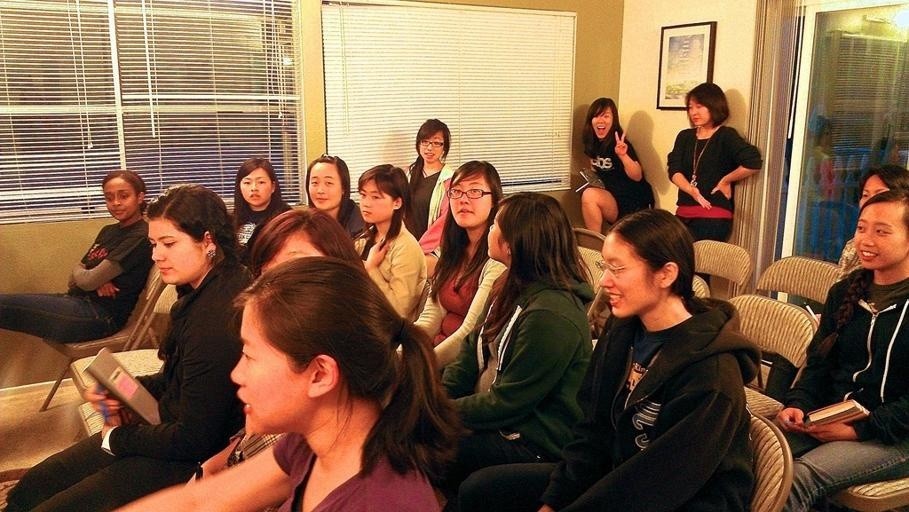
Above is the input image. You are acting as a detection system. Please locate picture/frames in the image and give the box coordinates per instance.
[656,21,717,111]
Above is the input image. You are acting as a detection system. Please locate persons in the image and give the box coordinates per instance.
[4,183,255,512]
[771,189,909,511]
[420,191,596,483]
[667,84,762,288]
[458,208,762,511]
[579,97,655,237]
[0,170,155,343]
[833,165,909,282]
[809,114,837,202]
[229,120,504,373]
[119,255,458,512]
[239,209,366,467]
[853,138,900,203]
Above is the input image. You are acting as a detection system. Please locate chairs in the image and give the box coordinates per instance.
[47,346,167,431]
[756,254,843,317]
[723,289,822,416]
[687,273,712,301]
[571,226,616,338]
[692,237,753,298]
[43,254,167,409]
[846,476,909,510]
[740,411,795,512]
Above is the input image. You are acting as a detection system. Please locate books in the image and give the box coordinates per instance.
[807,399,870,425]
[575,168,605,194]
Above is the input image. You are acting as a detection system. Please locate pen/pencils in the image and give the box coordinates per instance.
[96,386,109,423]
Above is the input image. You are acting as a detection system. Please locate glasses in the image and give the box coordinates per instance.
[421,141,444,147]
[447,188,492,199]
[594,259,630,278]
